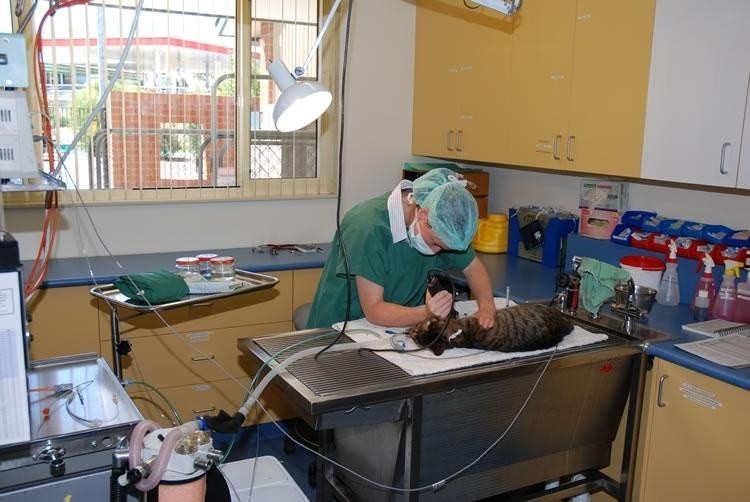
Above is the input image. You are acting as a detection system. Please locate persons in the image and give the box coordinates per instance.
[307,168,498,329]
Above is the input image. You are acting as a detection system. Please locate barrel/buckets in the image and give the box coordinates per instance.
[471,213,511,254]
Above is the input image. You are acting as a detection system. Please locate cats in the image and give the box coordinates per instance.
[407,304,574,356]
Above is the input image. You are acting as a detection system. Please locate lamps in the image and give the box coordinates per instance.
[266,0,524,134]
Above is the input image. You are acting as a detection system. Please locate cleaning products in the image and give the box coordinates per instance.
[690,250,715,315]
[732,271,750,325]
[655,238,680,306]
[712,258,746,321]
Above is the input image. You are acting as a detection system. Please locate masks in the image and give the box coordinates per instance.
[407,220,439,257]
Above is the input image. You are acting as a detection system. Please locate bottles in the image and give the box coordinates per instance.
[175,254,235,282]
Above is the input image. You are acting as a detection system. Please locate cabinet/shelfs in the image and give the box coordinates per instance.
[97,269,300,432]
[412,1,750,189]
[21,285,102,363]
[638,356,750,502]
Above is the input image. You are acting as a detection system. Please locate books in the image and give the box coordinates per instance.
[674,328,750,370]
[681,318,748,338]
[185,276,245,294]
